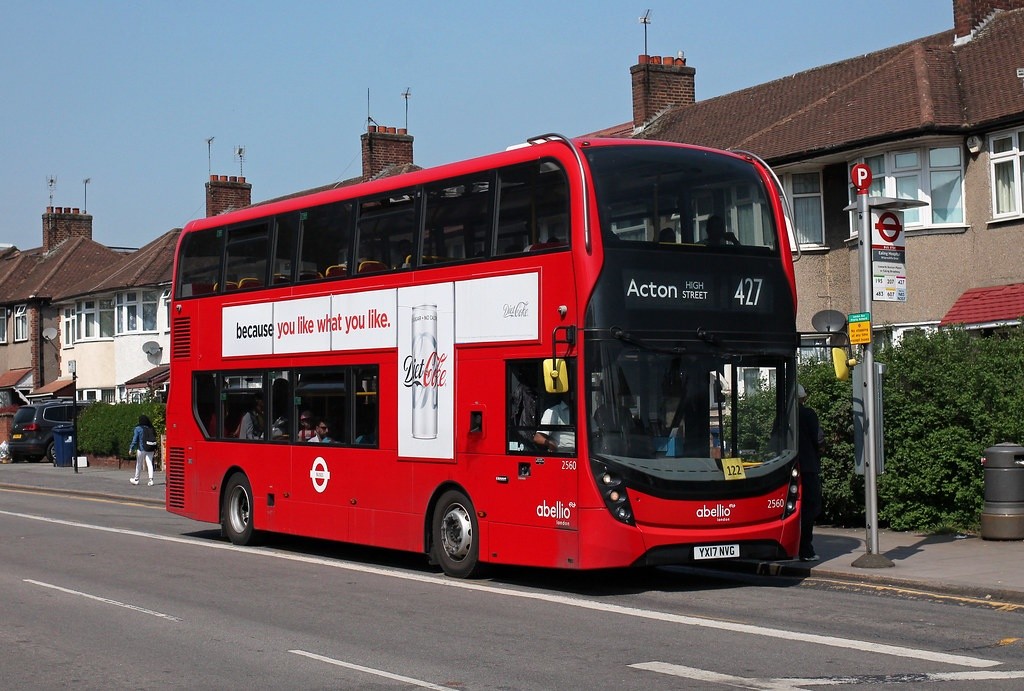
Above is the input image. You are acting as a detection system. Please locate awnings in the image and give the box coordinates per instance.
[125,366,170,400]
[0,368,33,405]
[26,381,74,398]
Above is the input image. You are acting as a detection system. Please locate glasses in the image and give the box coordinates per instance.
[319,426,330,430]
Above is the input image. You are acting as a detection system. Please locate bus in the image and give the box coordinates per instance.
[164,133,862,580]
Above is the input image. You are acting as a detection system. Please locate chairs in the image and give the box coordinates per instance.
[190,227,679,296]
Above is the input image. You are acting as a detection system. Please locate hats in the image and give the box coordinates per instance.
[300,411,313,420]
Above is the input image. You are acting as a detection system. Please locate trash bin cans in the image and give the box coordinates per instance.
[982,442,1024,539]
[52,425,75,466]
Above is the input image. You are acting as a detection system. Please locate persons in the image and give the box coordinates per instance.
[356,418,376,443]
[208,393,265,439]
[771,383,825,562]
[534,380,600,453]
[659,228,676,242]
[297,411,331,442]
[129,416,154,486]
[697,216,740,245]
[397,238,412,268]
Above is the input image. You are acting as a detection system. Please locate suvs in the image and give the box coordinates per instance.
[6,397,114,464]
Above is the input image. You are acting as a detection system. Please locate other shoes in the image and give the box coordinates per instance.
[130,477,139,485]
[148,481,154,487]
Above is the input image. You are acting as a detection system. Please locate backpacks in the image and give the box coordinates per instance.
[140,425,157,452]
